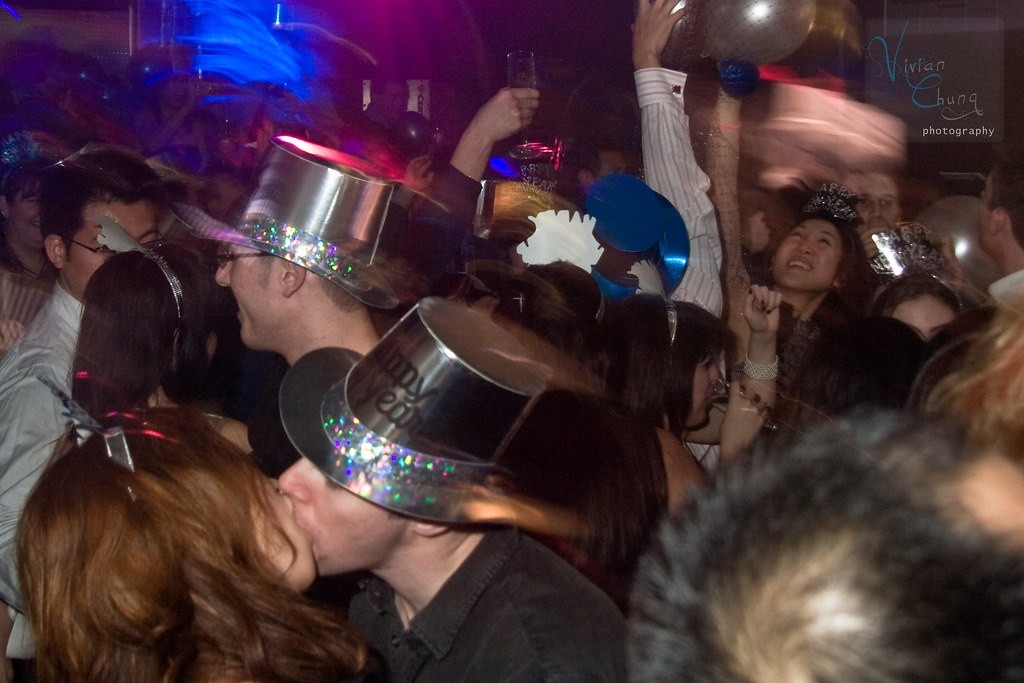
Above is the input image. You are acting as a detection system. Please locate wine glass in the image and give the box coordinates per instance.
[506,50,541,160]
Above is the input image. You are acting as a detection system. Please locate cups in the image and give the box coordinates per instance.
[709,379,776,432]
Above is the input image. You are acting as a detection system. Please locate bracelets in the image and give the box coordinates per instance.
[744,352,778,380]
[740,385,776,430]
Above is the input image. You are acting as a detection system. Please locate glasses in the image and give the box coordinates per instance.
[210,252,278,270]
[62,230,166,255]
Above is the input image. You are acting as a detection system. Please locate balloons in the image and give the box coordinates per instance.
[634,0,865,66]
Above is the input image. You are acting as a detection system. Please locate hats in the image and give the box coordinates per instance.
[278,296,586,538]
[164,135,411,309]
[572,171,690,308]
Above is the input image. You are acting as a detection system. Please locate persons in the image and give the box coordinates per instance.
[708,59,871,366]
[0,80,1024,683]
[576,0,725,469]
